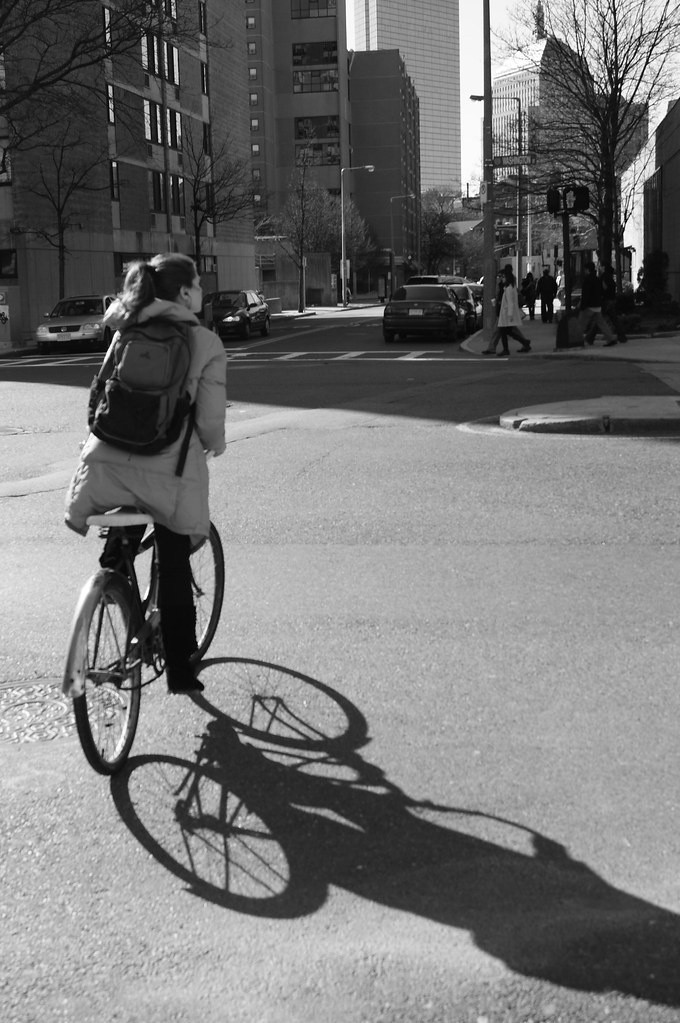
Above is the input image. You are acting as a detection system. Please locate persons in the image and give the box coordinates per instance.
[346,287,352,304]
[537,270,557,322]
[582,263,627,344]
[521,272,539,320]
[482,270,532,357]
[66,252,226,693]
[556,270,566,307]
[575,261,618,347]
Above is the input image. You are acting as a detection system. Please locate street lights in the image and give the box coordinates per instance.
[390,194,415,296]
[341,166,374,308]
[470,96,522,295]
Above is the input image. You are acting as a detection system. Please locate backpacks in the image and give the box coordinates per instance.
[86,314,200,456]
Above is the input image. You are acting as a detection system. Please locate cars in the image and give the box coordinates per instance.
[383,275,484,343]
[35,294,117,353]
[194,289,271,338]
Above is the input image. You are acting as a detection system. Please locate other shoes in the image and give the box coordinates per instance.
[526,340,531,351]
[585,336,593,345]
[164,665,204,695]
[99,594,116,607]
[603,340,617,346]
[497,350,509,356]
[517,346,529,352]
[482,350,496,355]
[617,337,627,343]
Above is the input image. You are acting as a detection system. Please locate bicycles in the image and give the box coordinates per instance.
[61,448,225,776]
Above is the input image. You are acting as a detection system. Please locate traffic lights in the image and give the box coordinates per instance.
[563,187,590,210]
[546,190,561,214]
[554,246,558,258]
